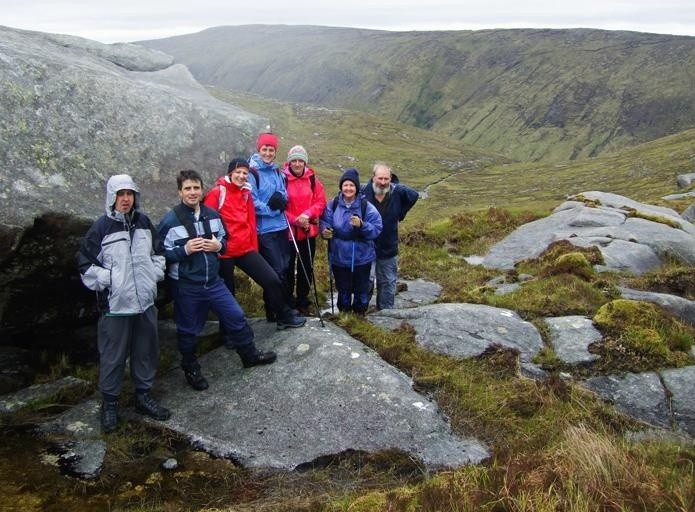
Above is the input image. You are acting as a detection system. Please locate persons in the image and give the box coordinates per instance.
[154,171,278,390]
[75,172,174,431]
[245,131,301,323]
[358,161,419,313]
[319,166,385,313]
[196,158,309,349]
[280,145,325,318]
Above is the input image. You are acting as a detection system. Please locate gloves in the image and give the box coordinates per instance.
[267,192,287,212]
[351,217,362,228]
[322,228,333,238]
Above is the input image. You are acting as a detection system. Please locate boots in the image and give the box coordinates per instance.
[180,360,208,391]
[135,392,170,420]
[235,342,277,368]
[100,400,120,432]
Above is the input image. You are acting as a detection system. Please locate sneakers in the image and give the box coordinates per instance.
[276,317,306,330]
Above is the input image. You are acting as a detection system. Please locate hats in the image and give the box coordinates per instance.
[228,158,249,177]
[339,169,360,191]
[287,145,309,164]
[257,133,279,154]
